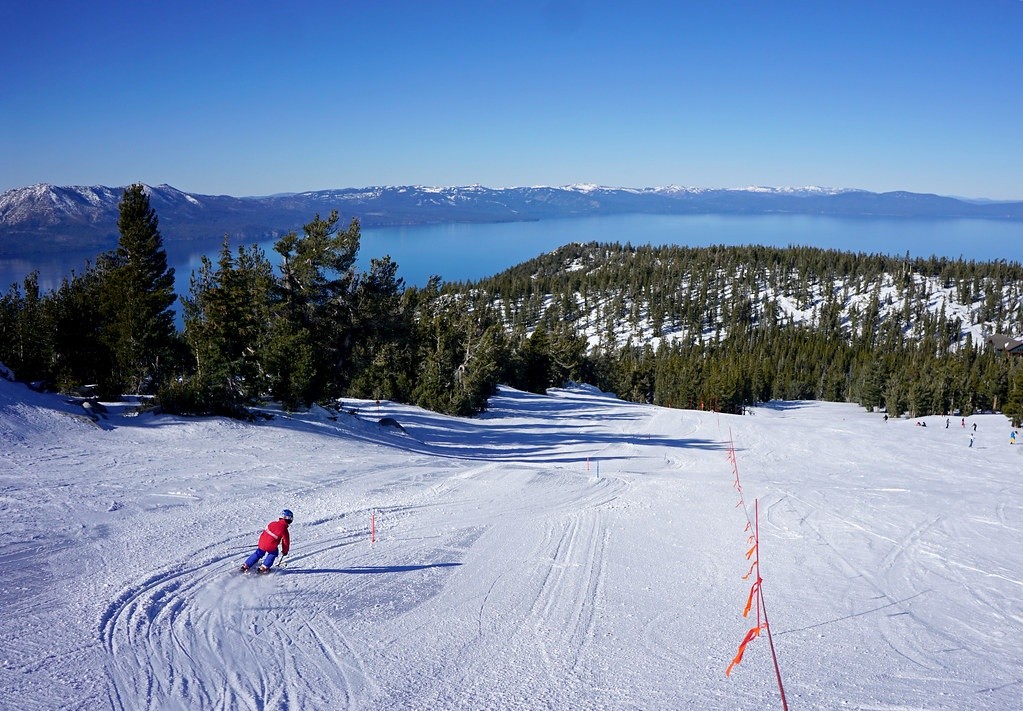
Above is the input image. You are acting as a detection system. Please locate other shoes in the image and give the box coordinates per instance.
[257,564,270,574]
[240,564,249,572]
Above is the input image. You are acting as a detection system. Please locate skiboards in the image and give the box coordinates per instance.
[230,562,287,578]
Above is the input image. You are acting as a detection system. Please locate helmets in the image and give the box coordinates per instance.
[279,509,294,523]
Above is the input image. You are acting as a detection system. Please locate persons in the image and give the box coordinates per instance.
[962,418,965,428]
[883,415,888,421]
[239,509,293,574]
[972,423,977,431]
[1010,431,1015,444]
[969,434,974,447]
[916,422,926,426]
[946,419,949,428]
